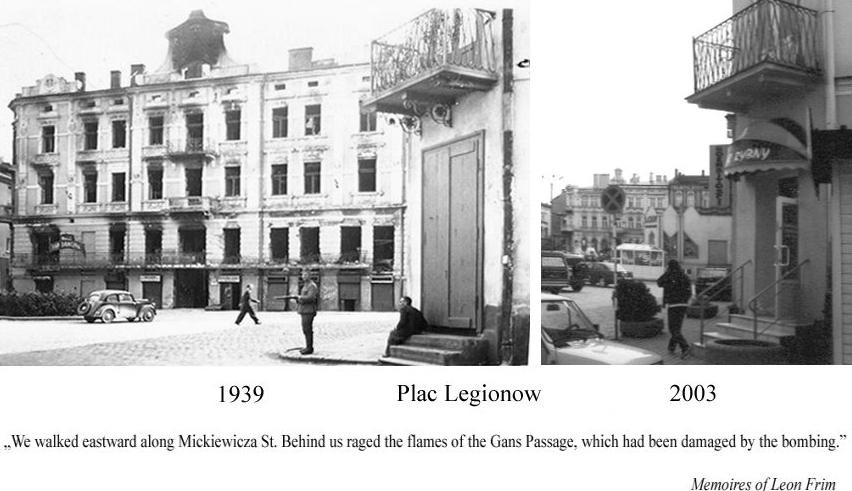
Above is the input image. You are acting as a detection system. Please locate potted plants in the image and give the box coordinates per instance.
[611,276,665,343]
[686,294,719,320]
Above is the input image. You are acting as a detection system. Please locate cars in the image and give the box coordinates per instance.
[541,291,664,364]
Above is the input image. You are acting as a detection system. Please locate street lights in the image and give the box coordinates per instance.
[541,173,566,199]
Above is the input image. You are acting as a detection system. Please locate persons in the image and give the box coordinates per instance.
[234,284,262,326]
[382,296,428,357]
[656,259,692,359]
[297,270,319,355]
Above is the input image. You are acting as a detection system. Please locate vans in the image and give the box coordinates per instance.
[542,249,573,292]
[563,253,589,291]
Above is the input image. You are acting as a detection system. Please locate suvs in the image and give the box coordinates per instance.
[592,261,634,287]
[77,289,157,322]
